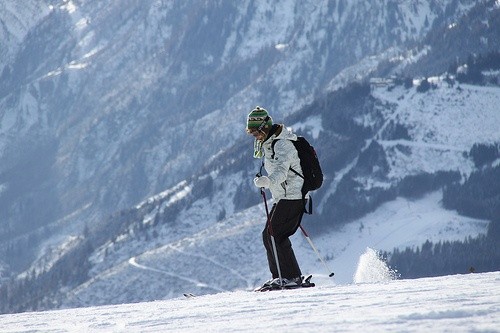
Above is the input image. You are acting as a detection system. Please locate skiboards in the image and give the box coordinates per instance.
[181,273,317,298]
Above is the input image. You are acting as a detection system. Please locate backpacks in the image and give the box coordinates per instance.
[271,136,323,191]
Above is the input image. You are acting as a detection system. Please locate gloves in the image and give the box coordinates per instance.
[253,176,270,187]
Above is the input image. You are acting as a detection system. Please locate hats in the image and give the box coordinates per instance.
[247,106,273,158]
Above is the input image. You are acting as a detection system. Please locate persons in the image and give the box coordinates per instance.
[247,106,309,288]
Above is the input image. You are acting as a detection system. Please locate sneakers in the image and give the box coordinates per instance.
[262,279,273,287]
[271,275,303,287]
